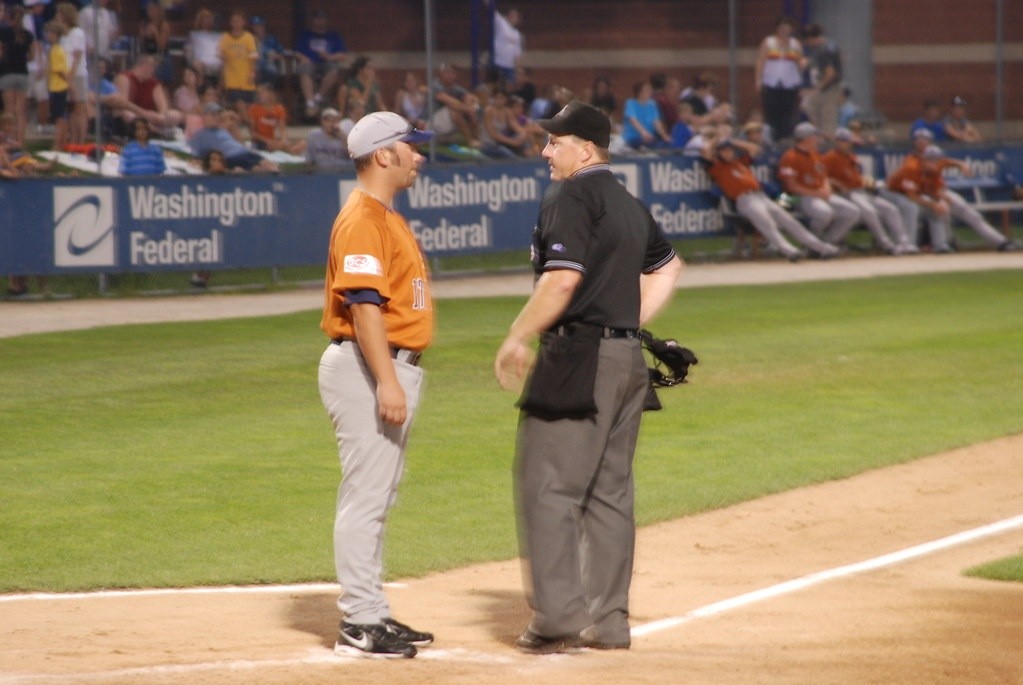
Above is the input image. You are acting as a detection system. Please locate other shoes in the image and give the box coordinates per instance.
[514,626,579,654]
[787,240,1019,262]
[573,633,631,650]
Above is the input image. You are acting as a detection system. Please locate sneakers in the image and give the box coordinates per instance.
[380,617,434,648]
[333,620,418,661]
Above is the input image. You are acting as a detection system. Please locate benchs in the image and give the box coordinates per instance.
[722,200,1022,258]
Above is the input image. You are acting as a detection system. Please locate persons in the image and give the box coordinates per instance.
[907,128,1014,252]
[881,145,953,253]
[910,97,980,144]
[620,17,876,153]
[494,100,680,657]
[591,78,621,134]
[317,112,435,658]
[700,125,850,261]
[822,128,919,255]
[393,0,573,159]
[0,0,389,295]
[779,122,859,260]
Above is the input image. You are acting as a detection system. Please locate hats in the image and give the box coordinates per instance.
[252,17,264,26]
[346,111,437,159]
[203,103,223,115]
[536,99,612,148]
[44,22,65,33]
[321,108,338,119]
[710,120,942,161]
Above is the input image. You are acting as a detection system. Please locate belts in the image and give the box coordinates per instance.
[540,319,640,339]
[330,335,422,368]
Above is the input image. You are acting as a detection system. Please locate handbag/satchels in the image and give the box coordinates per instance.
[515,335,600,421]
